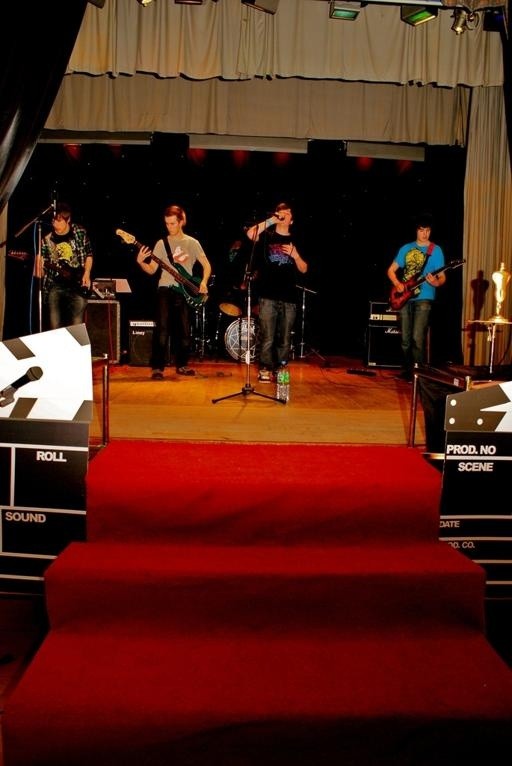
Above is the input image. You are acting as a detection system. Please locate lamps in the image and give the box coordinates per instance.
[238,0,510,34]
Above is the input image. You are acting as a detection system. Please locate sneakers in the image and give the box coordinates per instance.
[395,372,414,383]
[258,370,271,383]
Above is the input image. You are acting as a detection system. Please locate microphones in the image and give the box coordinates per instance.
[1,366,43,400]
[52,184,59,218]
[272,211,286,220]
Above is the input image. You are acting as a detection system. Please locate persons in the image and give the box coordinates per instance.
[229,222,261,316]
[246,203,307,384]
[387,220,446,381]
[136,205,212,380]
[34,203,93,330]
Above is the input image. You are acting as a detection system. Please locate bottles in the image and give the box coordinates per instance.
[276,360,290,402]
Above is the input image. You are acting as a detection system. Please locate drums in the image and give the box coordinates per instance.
[219,289,243,317]
[224,317,259,364]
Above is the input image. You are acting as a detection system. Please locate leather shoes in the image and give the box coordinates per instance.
[176,366,194,375]
[152,369,163,379]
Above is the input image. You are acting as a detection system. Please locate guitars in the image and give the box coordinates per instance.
[390,258,467,309]
[6,249,97,300]
[115,228,211,308]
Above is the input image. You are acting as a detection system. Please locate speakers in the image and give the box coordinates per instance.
[128,320,171,366]
[366,297,405,369]
[84,300,120,364]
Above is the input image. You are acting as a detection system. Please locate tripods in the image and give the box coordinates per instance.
[212,220,289,406]
[290,285,330,367]
